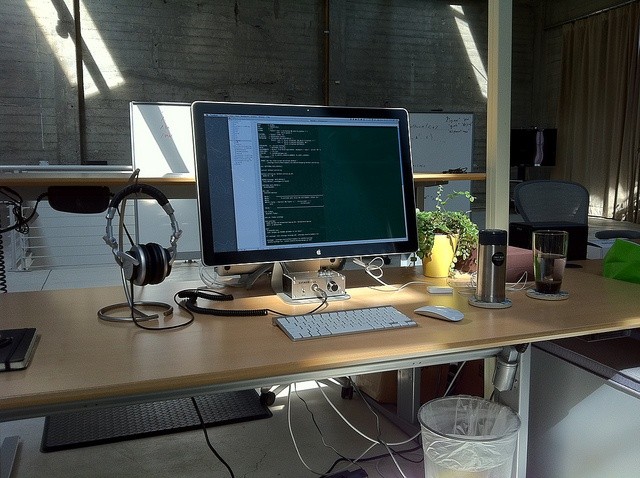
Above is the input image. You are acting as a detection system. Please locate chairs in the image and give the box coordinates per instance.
[515,180,589,224]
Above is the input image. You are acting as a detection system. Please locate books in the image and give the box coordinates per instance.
[1,328,39,373]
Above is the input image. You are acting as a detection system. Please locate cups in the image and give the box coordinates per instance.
[532,229,569,295]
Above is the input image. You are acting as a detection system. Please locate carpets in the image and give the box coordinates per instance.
[40,389,274,454]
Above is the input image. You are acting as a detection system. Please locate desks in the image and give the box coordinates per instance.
[0,265,639,384]
[0,173,488,204]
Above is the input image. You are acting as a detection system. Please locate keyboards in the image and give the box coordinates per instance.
[273,306,418,341]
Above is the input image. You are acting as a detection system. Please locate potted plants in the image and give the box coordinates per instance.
[407,184,480,278]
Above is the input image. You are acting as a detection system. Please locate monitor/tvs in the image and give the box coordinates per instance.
[190,100,419,305]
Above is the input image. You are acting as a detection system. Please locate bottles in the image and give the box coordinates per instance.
[475,229,507,303]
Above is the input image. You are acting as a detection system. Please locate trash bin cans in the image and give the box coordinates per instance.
[417,395,521,478]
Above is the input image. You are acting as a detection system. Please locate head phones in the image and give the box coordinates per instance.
[103,184,183,286]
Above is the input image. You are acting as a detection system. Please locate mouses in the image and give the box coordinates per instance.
[414,306,464,322]
